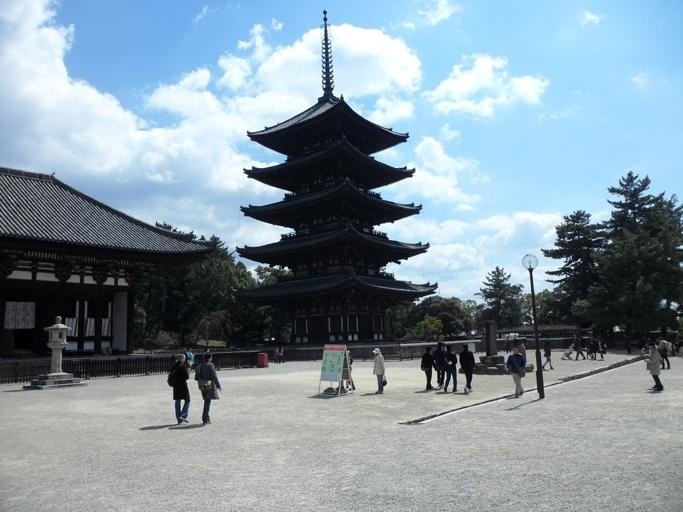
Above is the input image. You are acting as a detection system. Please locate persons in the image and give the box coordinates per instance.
[187,346,195,374]
[505,345,526,398]
[441,344,458,393]
[335,345,356,391]
[560,332,607,364]
[193,351,221,424]
[623,330,683,370]
[442,343,446,354]
[419,345,435,390]
[170,353,191,425]
[430,342,446,385]
[371,348,385,393]
[183,347,190,376]
[459,344,475,394]
[270,344,284,364]
[540,340,553,371]
[643,342,664,391]
[517,342,527,365]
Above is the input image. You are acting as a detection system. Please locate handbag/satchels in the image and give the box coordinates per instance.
[519,368,525,377]
[380,376,386,386]
[197,379,212,391]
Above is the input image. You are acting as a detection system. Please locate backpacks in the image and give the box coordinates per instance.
[167,369,176,386]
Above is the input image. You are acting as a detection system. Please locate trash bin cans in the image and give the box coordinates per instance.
[258,353,268,368]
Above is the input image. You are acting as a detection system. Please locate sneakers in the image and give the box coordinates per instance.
[426,384,473,395]
[178,417,189,424]
[654,384,662,390]
[203,419,211,424]
[515,392,523,398]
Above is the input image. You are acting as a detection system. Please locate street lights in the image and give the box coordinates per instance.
[521,254,545,399]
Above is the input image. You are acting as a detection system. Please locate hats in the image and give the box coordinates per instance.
[372,347,381,354]
